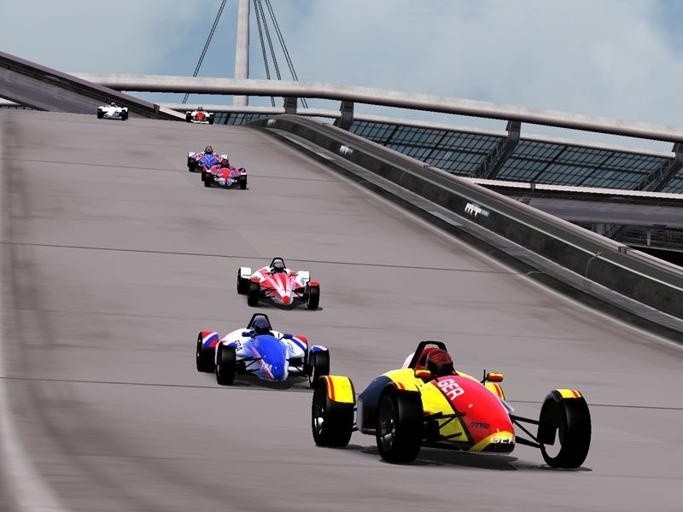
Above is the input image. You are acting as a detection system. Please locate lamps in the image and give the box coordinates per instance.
[407,341,454,370]
[270,257,286,268]
[246,312,273,329]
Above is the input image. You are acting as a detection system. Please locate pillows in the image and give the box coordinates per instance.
[425,349,453,378]
[254,318,270,334]
[274,262,284,273]
[221,159,228,167]
[206,146,212,153]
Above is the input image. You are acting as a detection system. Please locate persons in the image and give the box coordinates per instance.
[109,102,118,107]
[216,158,229,168]
[197,105,203,111]
[251,316,274,338]
[425,349,458,383]
[203,145,214,155]
[265,256,287,274]
